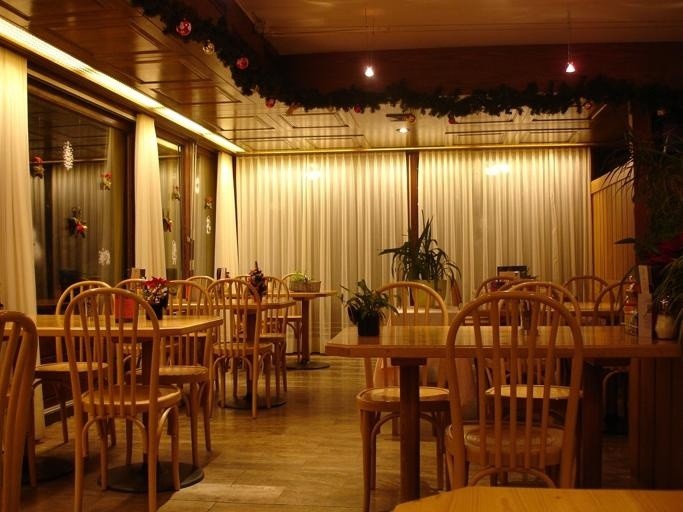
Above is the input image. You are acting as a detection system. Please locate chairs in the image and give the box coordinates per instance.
[230,275,252,294]
[204,279,273,419]
[482,278,560,389]
[64,288,181,512]
[34,280,117,446]
[113,278,163,296]
[265,273,308,362]
[358,281,452,512]
[559,274,615,326]
[445,289,583,492]
[232,276,290,399]
[125,279,215,467]
[177,276,218,302]
[593,280,631,426]
[0,310,39,512]
[489,281,583,487]
[474,277,516,301]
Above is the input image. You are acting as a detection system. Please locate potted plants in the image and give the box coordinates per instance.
[337,279,401,337]
[601,109,683,323]
[377,208,462,308]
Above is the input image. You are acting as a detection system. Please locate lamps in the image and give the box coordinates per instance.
[565,11,576,73]
[396,126,409,134]
[365,16,375,78]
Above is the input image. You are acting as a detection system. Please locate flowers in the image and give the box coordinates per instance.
[142,276,175,303]
[248,260,267,295]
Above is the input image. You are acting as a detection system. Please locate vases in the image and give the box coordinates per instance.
[253,292,263,303]
[147,303,163,320]
